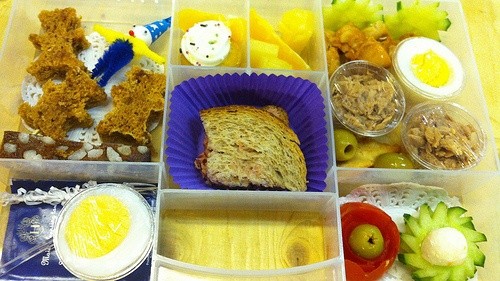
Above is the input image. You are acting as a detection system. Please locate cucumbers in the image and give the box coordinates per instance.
[395,201,487,281]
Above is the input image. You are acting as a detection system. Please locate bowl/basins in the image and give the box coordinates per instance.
[401,100,487,171]
[52,182,156,281]
[387,36,466,113]
[329,59,406,137]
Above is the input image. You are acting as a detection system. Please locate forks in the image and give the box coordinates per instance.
[0,182,158,279]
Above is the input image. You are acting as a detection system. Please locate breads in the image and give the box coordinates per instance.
[97,65,166,147]
[16,67,106,139]
[27,8,91,85]
[194,104,309,191]
